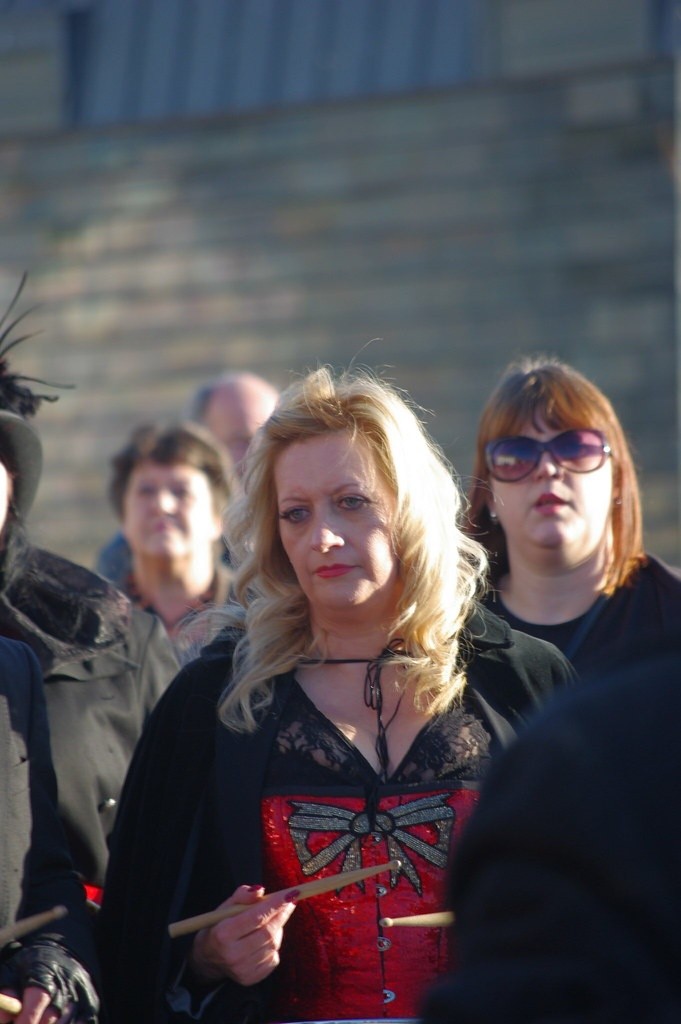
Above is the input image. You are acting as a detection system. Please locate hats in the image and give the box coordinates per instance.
[1,271,74,523]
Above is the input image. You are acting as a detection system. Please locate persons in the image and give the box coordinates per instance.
[412,629,681,1024]
[99,372,312,724]
[105,369,579,1022]
[1,349,181,1024]
[95,418,290,777]
[441,351,681,796]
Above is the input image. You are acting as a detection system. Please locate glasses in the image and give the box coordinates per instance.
[486,429,612,481]
